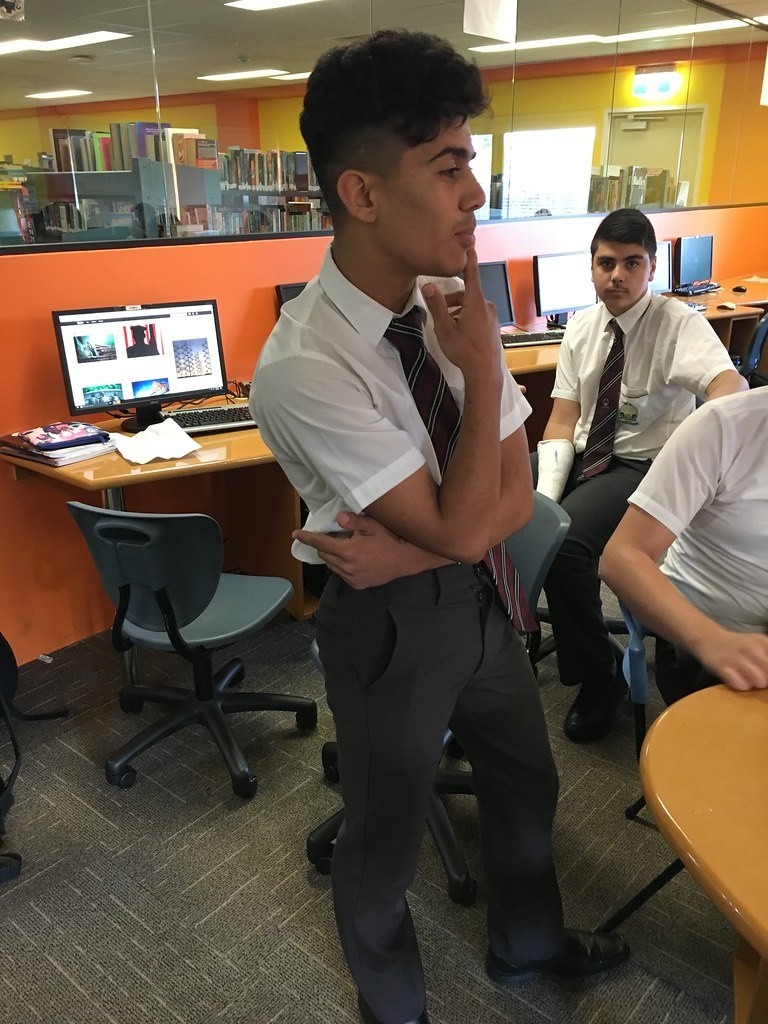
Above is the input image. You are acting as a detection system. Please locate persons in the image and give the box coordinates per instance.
[130,203,172,238]
[535,208,750,745]
[599,381,768,707]
[247,27,629,1024]
[244,210,272,234]
[21,202,85,243]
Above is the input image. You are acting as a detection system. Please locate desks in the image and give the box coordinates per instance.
[640,682,768,1024]
[0,275,768,696]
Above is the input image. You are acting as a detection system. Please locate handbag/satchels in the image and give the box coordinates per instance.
[20,420,110,451]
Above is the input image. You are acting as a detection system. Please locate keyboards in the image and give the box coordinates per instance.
[501,331,566,348]
[163,403,257,435]
[674,283,721,296]
[684,302,707,311]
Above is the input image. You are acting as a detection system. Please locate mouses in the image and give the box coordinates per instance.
[733,286,746,292]
[716,302,739,310]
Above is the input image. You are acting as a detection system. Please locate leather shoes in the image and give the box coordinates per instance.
[564,652,627,739]
[357,991,430,1024]
[486,928,629,981]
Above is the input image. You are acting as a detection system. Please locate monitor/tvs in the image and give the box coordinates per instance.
[533,251,599,329]
[455,261,516,327]
[675,235,713,288]
[51,299,229,434]
[276,282,308,310]
[648,241,673,293]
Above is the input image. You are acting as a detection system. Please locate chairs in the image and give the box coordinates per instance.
[67,313,768,976]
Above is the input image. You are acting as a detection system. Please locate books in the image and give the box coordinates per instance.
[0,433,118,468]
[173,196,335,237]
[48,123,219,172]
[111,199,135,212]
[216,146,323,192]
[586,164,690,213]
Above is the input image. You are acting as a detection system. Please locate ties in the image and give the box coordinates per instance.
[383,306,538,633]
[581,318,624,479]
